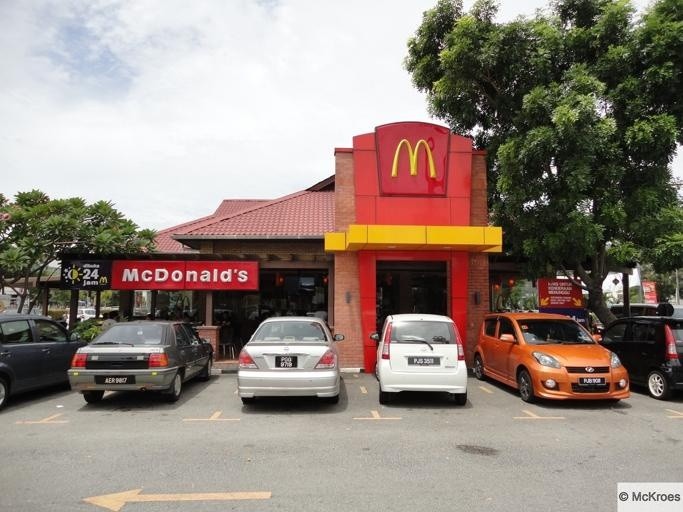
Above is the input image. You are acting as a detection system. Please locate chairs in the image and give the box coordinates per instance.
[219,327,235,358]
[264,336,319,341]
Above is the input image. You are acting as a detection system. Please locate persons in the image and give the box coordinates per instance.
[248,304,328,322]
[213,309,236,326]
[145,307,200,323]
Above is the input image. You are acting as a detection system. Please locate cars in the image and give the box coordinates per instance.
[0,312,88,410]
[65,317,215,406]
[473,311,631,406]
[62,299,121,329]
[369,313,468,406]
[598,318,682,399]
[238,315,346,404]
[610,303,682,317]
